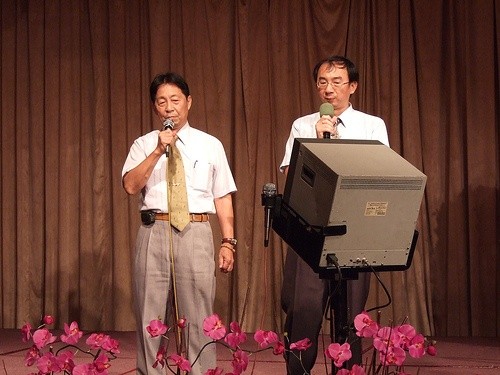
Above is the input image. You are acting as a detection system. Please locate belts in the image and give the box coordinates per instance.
[154,213,210,223]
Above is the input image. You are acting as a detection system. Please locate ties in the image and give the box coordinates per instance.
[331,118,341,140]
[167,135,190,231]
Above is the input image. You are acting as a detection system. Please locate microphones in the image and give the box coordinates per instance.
[162,119,174,158]
[263,182,276,247]
[319,103,334,139]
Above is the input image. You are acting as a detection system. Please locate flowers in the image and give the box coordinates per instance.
[22,309,438,375]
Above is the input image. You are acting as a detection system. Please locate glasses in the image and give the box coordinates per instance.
[317,79,350,90]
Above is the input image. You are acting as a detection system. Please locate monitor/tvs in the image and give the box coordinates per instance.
[283,137,427,268]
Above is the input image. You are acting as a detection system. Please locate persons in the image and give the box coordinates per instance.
[278,57,390,375]
[121,73,237,375]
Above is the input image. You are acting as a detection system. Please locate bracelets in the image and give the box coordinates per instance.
[221,245,235,252]
[222,238,237,245]
